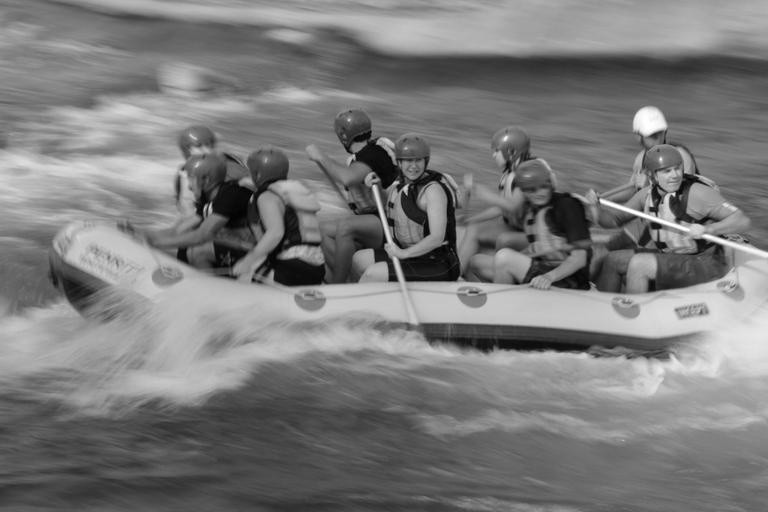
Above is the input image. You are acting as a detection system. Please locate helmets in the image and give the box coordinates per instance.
[334,109,372,150]
[182,153,227,194]
[246,147,289,191]
[515,158,555,192]
[491,125,530,166]
[180,125,216,158]
[394,133,431,168]
[632,105,668,139]
[642,144,684,187]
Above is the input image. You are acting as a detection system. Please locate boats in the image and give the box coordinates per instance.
[46,214,768,354]
